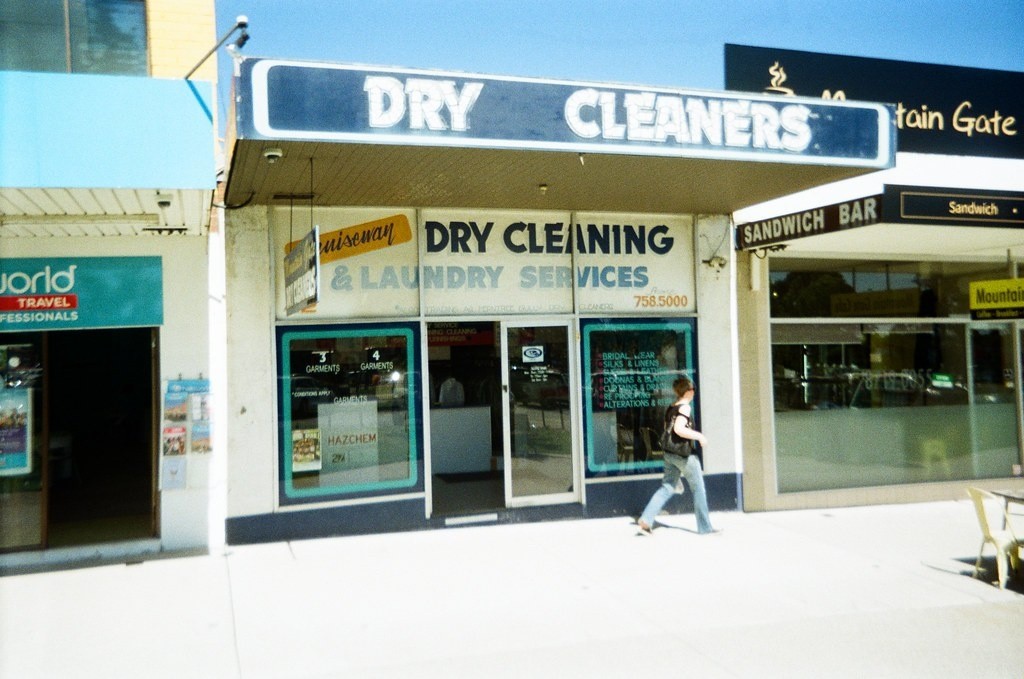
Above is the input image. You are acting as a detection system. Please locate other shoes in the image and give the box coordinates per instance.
[633,525,655,537]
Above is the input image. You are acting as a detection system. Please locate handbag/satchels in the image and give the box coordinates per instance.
[657,409,693,458]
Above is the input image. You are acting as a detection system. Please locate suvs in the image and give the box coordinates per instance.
[589,370,699,411]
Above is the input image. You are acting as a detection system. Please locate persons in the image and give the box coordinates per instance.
[634,377,716,534]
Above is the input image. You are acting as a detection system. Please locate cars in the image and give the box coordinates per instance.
[510,366,569,408]
[846,370,943,407]
[279,373,338,418]
